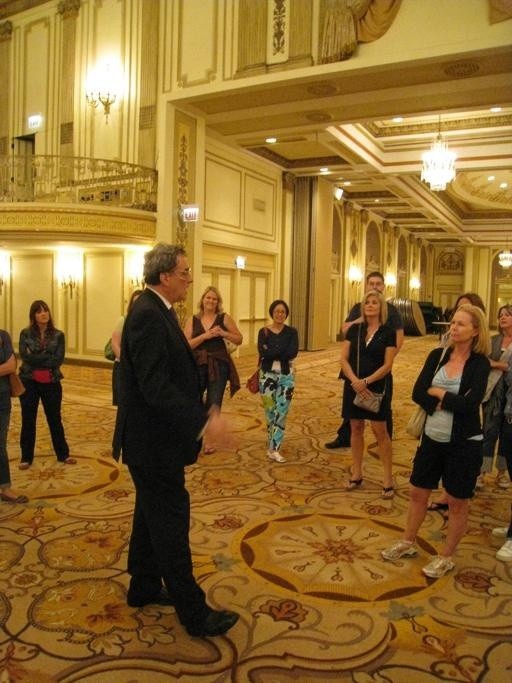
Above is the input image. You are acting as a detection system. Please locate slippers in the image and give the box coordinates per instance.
[382,483,394,499]
[343,477,363,491]
[203,441,216,454]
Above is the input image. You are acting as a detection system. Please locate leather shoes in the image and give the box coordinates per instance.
[186,610,240,638]
[325,436,350,448]
[66,458,77,464]
[0,492,29,504]
[19,463,29,470]
[125,585,173,609]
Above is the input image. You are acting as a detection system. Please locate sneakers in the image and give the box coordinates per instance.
[492,528,510,537]
[475,475,485,489]
[496,539,512,561]
[424,554,455,578]
[381,539,418,560]
[496,472,510,489]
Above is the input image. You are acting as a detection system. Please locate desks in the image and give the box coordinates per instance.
[433,321,450,340]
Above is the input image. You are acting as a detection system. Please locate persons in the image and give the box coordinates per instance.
[110,289,141,360]
[476,305,512,489]
[382,307,491,578]
[340,289,396,500]
[19,300,74,466]
[0,329,30,503]
[491,421,512,561]
[112,241,239,638]
[325,271,405,447]
[427,293,486,509]
[183,287,243,454]
[258,301,298,462]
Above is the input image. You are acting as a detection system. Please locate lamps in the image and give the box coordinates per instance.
[421,115,457,191]
[54,244,79,299]
[499,233,512,269]
[85,55,124,124]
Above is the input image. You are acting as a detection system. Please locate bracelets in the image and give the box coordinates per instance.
[364,377,368,383]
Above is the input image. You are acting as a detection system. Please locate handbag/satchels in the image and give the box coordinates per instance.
[221,339,238,355]
[245,372,259,393]
[9,373,26,398]
[104,317,127,361]
[354,387,384,413]
[406,405,428,439]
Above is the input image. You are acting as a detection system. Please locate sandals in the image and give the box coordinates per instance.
[268,451,286,463]
[428,498,448,510]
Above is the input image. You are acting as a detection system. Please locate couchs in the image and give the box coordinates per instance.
[418,302,442,332]
